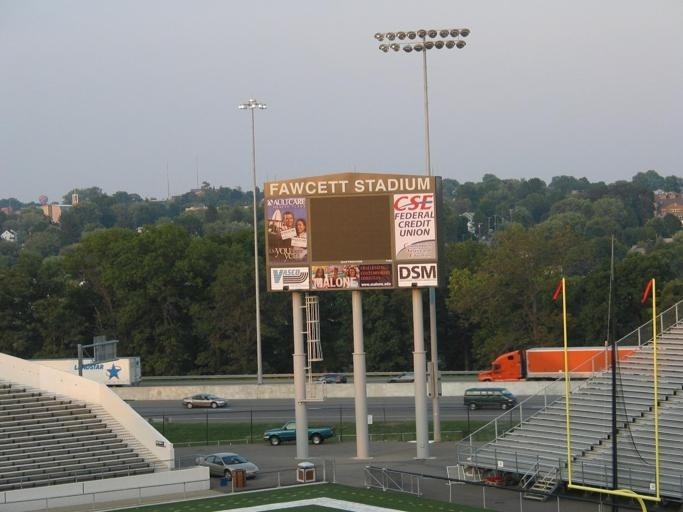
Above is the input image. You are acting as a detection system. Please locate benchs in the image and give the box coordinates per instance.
[459,316,683,501]
[1,383,157,491]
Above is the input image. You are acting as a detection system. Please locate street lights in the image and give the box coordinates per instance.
[372,25,473,439]
[237,96,273,385]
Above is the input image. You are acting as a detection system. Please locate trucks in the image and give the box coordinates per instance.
[477,346,647,381]
[26,355,143,390]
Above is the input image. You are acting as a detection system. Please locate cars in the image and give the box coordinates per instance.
[193,450,257,482]
[318,372,346,385]
[386,370,429,383]
[181,392,228,409]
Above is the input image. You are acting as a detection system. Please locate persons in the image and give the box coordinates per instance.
[328,266,341,285]
[345,267,358,287]
[293,218,306,237]
[313,267,324,279]
[343,268,350,277]
[281,211,294,228]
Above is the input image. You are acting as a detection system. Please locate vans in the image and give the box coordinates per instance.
[461,386,517,412]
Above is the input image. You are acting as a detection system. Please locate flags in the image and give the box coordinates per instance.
[637,278,653,304]
[551,277,565,302]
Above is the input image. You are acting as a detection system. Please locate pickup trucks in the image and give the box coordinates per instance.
[263,420,336,445]
